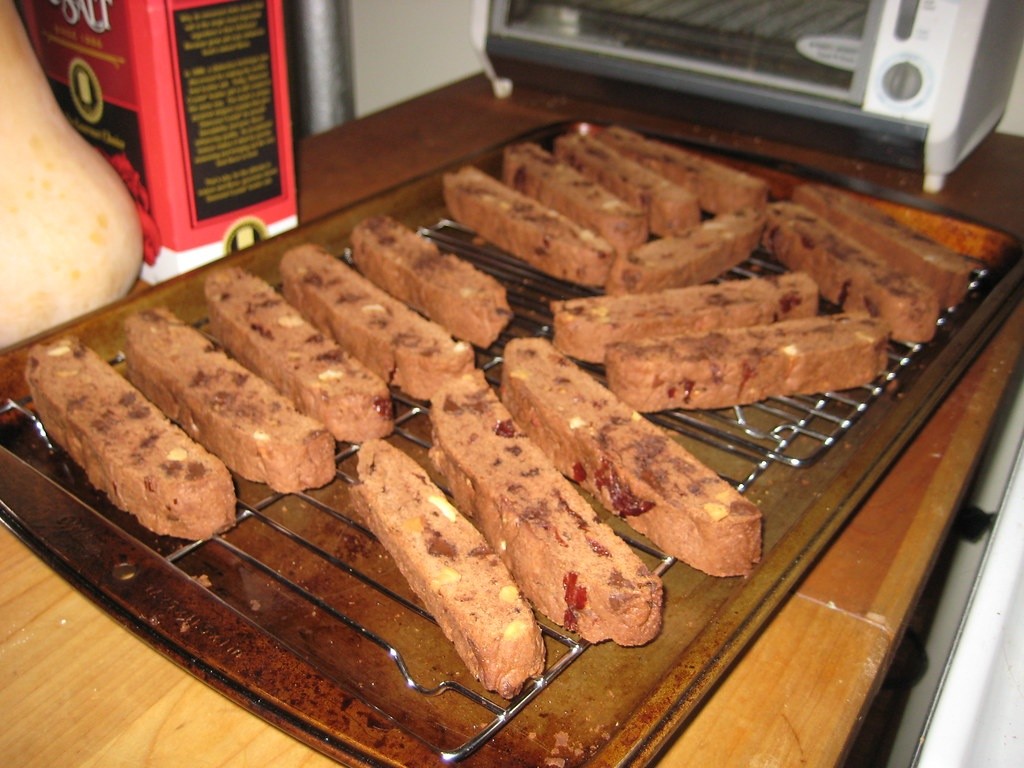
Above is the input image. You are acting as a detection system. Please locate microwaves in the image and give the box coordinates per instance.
[472,0,1024,191]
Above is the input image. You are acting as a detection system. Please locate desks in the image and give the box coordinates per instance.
[0,70,1024,768]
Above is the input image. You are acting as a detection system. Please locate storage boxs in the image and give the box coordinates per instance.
[13,0,300,286]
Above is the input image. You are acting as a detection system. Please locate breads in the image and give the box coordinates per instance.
[23,124,976,700]
